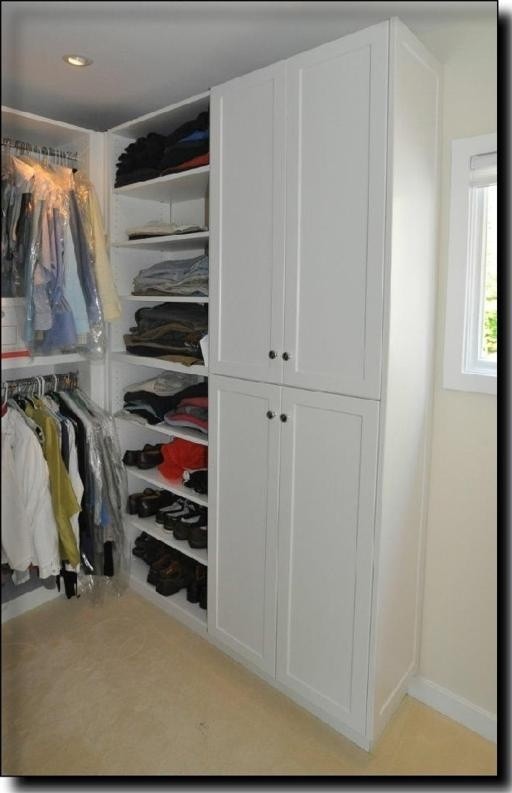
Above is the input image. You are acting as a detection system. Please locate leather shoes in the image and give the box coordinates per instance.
[137,443,165,469]
[133,532,207,608]
[129,487,208,548]
[123,443,155,465]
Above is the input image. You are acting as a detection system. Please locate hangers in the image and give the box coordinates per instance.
[3,136,94,194]
[1,371,114,445]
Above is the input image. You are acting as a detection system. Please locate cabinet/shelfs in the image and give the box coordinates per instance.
[3,106,103,623]
[102,89,209,638]
[219,43,373,381]
[217,375,377,714]
